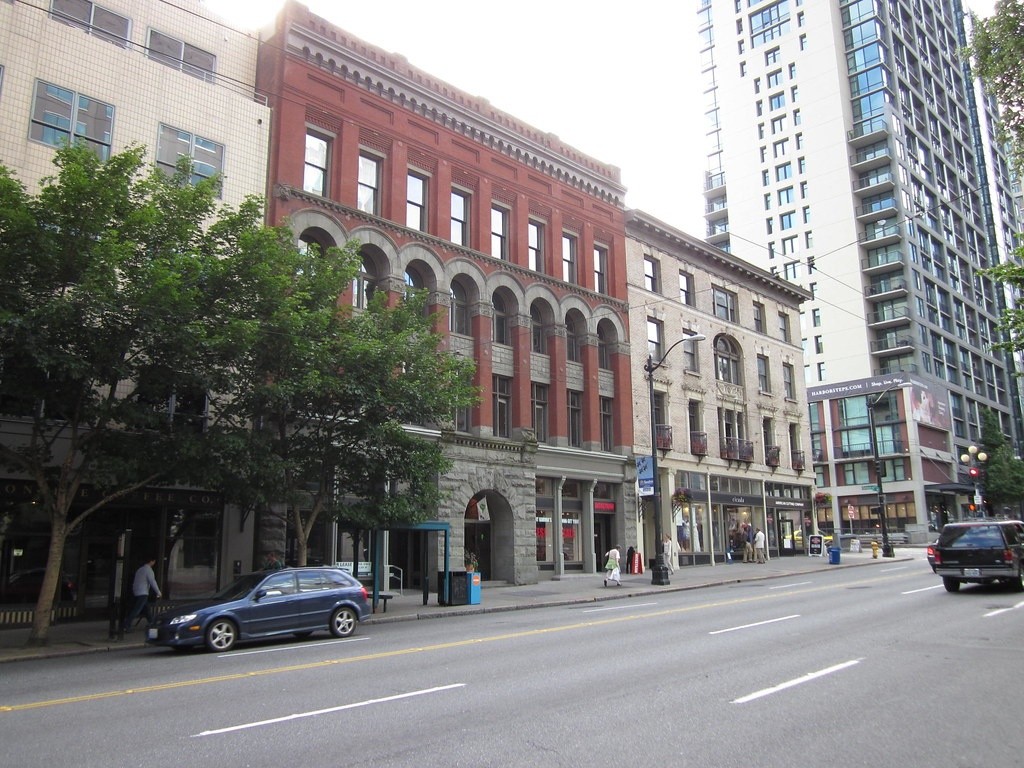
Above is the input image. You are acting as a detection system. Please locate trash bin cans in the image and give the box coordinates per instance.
[438,569,467,606]
[826,546,843,564]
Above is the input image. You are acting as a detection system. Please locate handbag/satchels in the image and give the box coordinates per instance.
[605,560,619,570]
[611,567,620,582]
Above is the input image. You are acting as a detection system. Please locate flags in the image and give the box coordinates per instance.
[969,467,979,477]
[474,495,492,522]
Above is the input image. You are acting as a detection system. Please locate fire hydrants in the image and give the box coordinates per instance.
[871,541,879,559]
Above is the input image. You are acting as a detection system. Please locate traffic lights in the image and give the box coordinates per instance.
[979,468,985,484]
[970,468,978,476]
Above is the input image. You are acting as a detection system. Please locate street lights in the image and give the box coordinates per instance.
[866,380,914,558]
[643,332,706,587]
[960,446,987,518]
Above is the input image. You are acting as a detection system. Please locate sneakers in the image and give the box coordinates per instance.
[604,580,607,587]
[617,583,622,585]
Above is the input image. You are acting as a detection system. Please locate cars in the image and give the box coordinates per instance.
[928,520,1024,593]
[145,564,373,654]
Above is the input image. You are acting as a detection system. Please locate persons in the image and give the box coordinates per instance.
[661,532,675,575]
[121,554,163,636]
[679,516,692,551]
[604,544,622,586]
[755,527,767,564]
[742,525,753,564]
[255,551,283,570]
